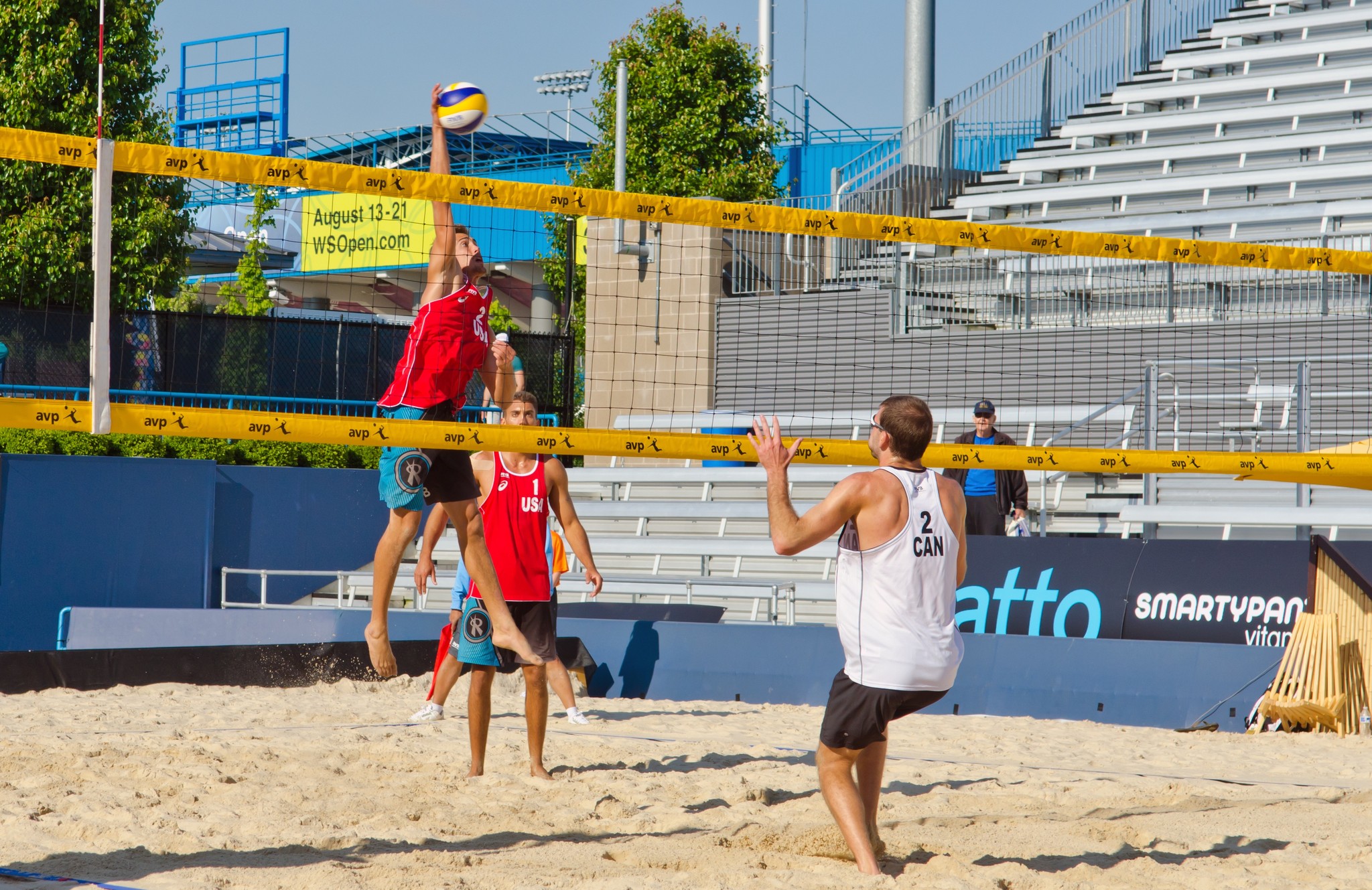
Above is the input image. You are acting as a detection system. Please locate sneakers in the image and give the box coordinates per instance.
[406,705,444,724]
[568,710,590,725]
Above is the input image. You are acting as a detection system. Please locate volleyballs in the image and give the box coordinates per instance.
[437,82,489,136]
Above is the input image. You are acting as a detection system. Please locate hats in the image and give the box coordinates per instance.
[973,399,995,413]
[495,332,509,342]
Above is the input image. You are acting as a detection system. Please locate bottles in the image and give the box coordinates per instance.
[1359,706,1371,739]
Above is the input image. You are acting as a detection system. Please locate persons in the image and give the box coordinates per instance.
[412,390,602,782]
[748,395,966,875]
[481,333,524,424]
[364,82,544,679]
[940,400,1028,534]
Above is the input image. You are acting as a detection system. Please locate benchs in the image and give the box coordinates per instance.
[823,0,1372,294]
[312,403,1372,625]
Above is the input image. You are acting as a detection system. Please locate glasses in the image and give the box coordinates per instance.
[975,413,992,419]
[871,413,893,435]
